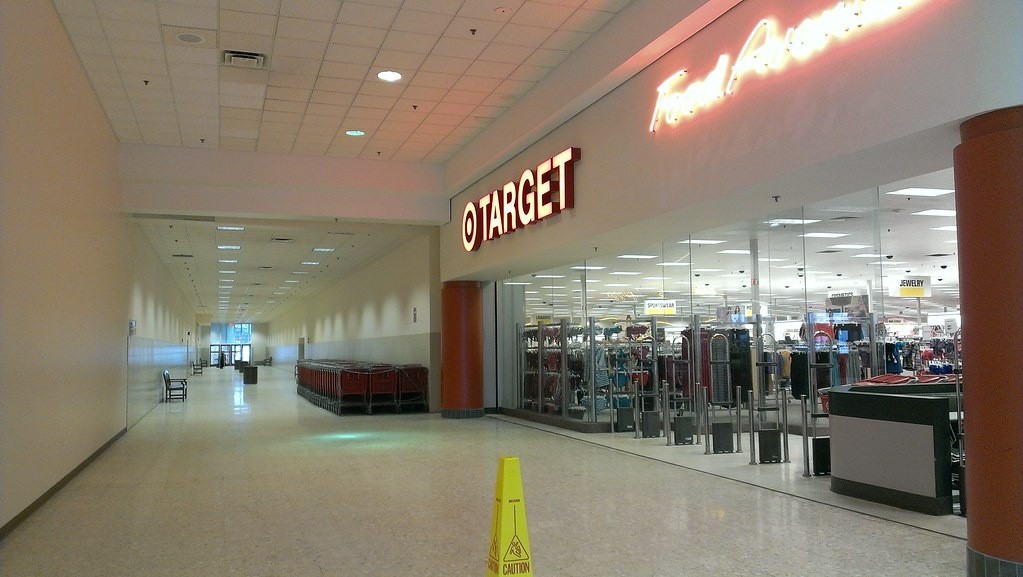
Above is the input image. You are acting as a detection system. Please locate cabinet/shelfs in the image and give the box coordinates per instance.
[517,317,660,422]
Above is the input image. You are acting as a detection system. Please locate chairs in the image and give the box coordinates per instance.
[263,357,272,366]
[193,362,202,375]
[200,358,207,367]
[163,370,188,402]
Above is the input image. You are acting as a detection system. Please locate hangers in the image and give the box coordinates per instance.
[929,358,954,368]
